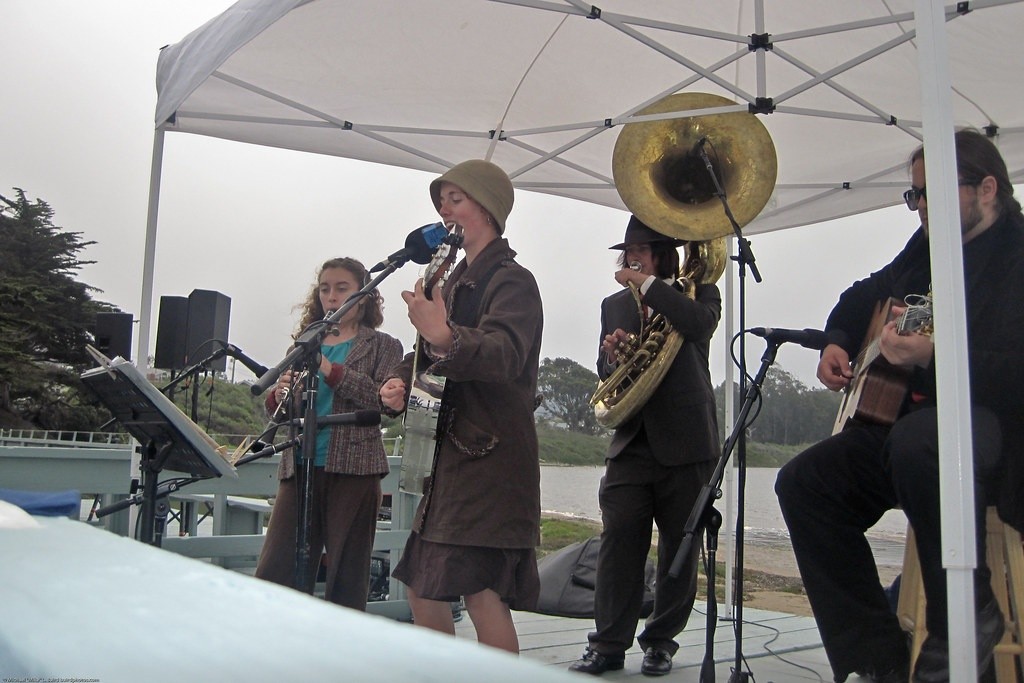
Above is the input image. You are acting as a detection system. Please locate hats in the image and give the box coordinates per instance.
[608,215,689,250]
[430,160,514,236]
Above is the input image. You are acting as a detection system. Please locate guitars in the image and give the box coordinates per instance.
[830,285,935,442]
[395,222,465,500]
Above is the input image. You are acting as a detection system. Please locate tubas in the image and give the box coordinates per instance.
[589,90,777,431]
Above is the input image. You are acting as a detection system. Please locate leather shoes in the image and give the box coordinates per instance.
[568,647,624,674]
[641,646,672,676]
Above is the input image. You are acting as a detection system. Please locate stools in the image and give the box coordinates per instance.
[892,504,1024,683]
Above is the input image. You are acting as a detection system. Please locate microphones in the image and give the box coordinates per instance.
[218,341,269,378]
[750,327,829,350]
[684,138,708,166]
[370,222,449,272]
[286,409,382,426]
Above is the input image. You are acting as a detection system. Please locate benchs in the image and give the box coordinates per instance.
[176,494,274,569]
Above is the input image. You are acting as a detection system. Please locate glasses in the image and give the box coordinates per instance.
[903,178,969,211]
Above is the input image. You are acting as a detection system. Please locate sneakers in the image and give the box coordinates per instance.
[855,633,912,683]
[911,597,1005,683]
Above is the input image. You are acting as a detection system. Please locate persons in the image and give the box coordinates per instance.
[566,216,723,676]
[253,258,404,613]
[775,122,1024,683]
[377,158,545,654]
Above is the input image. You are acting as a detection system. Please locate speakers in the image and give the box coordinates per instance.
[184,289,232,372]
[153,296,189,372]
[93,312,133,369]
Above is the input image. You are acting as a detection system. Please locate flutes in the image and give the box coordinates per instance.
[249,310,336,458]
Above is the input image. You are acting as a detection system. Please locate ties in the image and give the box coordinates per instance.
[638,289,648,319]
